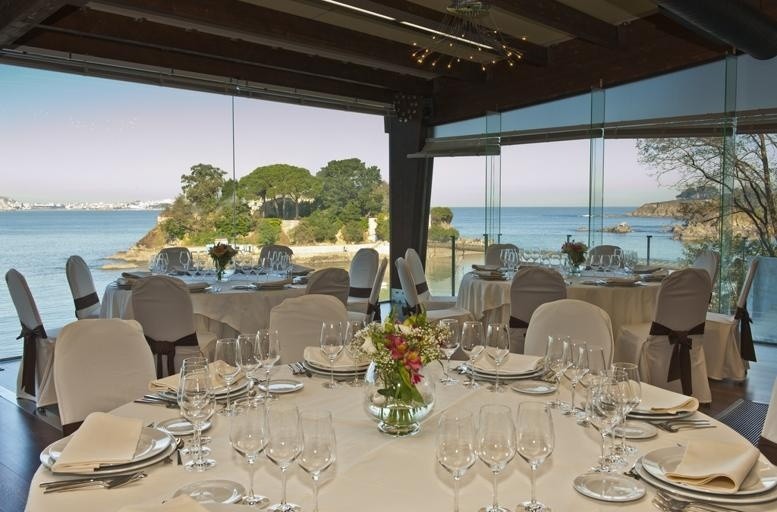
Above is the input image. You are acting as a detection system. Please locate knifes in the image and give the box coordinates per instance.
[288,360,312,379]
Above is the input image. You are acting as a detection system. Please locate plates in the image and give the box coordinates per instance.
[510,381,557,395]
[636,267,661,274]
[574,471,649,502]
[302,346,368,380]
[636,449,776,506]
[158,417,211,436]
[464,366,542,383]
[38,424,177,476]
[259,379,305,394]
[166,480,246,508]
[624,409,699,419]
[608,424,657,439]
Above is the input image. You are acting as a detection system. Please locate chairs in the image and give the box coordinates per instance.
[2,242,776,512]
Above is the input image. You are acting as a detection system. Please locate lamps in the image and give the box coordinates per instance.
[326,0,495,49]
[408,0,528,74]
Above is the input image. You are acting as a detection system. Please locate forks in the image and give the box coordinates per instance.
[39,472,146,494]
[645,418,717,432]
[651,489,742,512]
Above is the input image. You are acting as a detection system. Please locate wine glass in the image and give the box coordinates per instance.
[319,321,384,389]
[499,249,637,282]
[433,401,561,512]
[149,247,291,284]
[177,357,219,473]
[439,319,510,393]
[543,334,639,494]
[211,328,281,415]
[230,397,337,512]
